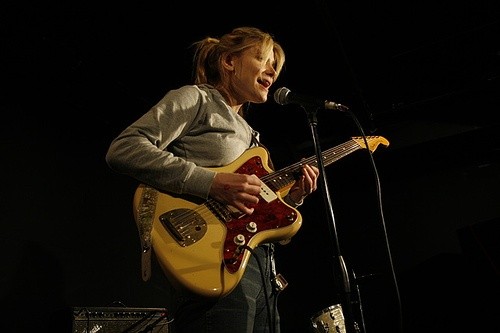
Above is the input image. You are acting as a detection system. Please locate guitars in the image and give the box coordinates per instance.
[133,136,389,299]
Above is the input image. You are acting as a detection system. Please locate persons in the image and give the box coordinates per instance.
[105,27,319,333]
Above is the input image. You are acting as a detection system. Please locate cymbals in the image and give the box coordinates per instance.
[337,272,381,294]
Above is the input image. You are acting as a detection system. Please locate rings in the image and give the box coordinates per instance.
[306,187,313,191]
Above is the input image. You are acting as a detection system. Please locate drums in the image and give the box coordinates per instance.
[309,302,359,333]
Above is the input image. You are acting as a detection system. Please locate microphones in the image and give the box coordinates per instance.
[274,87,348,111]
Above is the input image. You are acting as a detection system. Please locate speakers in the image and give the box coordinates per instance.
[72,306,176,333]
